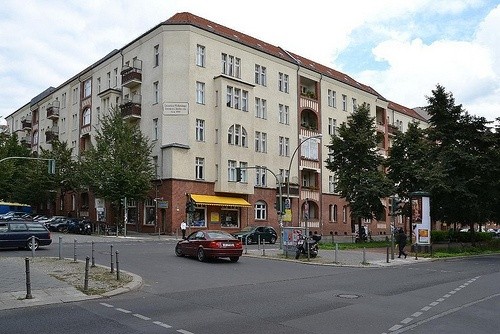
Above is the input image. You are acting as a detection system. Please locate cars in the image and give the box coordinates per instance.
[230,225,278,244]
[0,211,92,250]
[175,230,243,262]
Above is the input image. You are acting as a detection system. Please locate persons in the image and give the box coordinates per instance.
[181,220,187,239]
[396,229,408,258]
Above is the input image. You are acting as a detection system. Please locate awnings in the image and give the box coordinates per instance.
[190,194,252,208]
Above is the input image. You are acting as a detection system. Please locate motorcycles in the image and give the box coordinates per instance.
[295,233,322,259]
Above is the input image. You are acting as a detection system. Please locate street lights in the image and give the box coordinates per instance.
[231,165,283,249]
[287,135,322,198]
